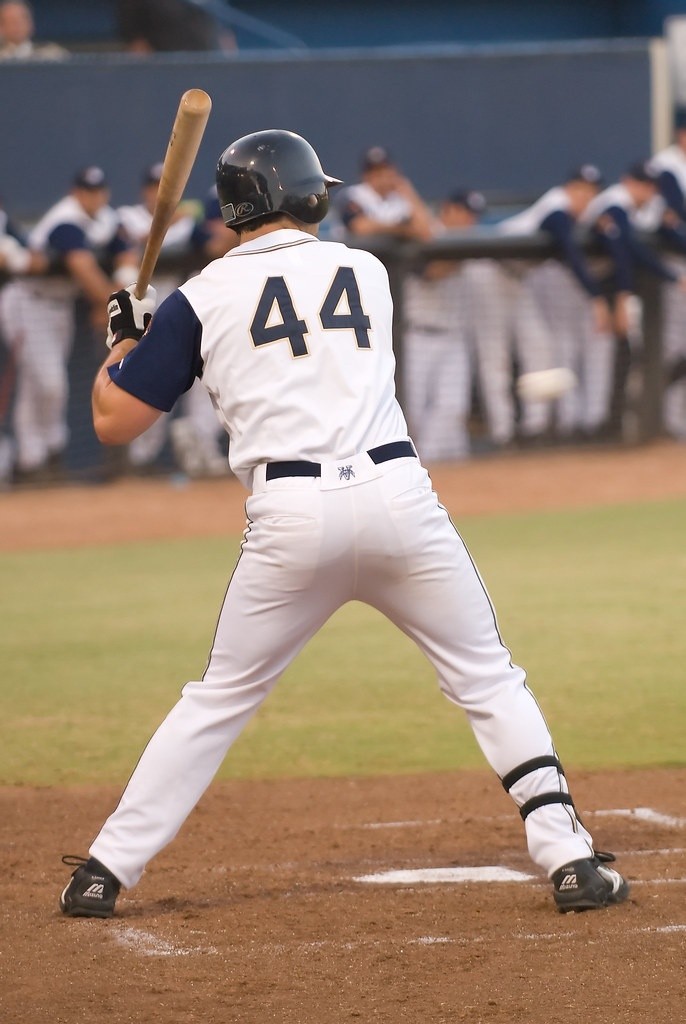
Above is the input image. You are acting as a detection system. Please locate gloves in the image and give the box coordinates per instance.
[106,283,158,352]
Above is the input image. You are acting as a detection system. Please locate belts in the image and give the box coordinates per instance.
[265,441,417,479]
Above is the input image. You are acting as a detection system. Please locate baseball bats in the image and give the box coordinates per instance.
[130,89,214,305]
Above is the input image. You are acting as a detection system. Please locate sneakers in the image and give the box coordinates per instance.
[552,851,628,912]
[59,853,120,918]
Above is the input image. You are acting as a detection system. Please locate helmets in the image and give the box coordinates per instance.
[216,129,344,228]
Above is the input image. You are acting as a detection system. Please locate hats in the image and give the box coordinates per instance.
[363,146,390,170]
[75,165,106,190]
[632,164,662,189]
[579,165,604,185]
[451,188,490,219]
[146,164,164,182]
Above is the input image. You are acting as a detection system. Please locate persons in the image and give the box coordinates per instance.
[56,128,627,920]
[0,1,686,487]
